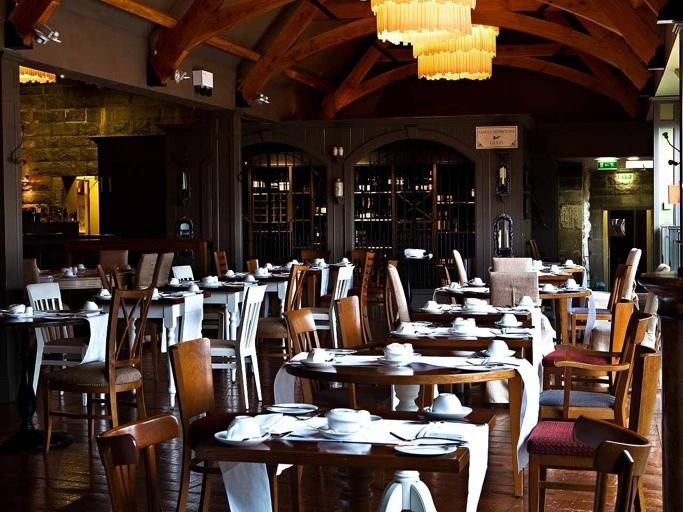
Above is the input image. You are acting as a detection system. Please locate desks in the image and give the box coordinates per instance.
[0,309,104,455]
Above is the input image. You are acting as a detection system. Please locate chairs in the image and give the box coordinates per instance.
[25,243,671,512]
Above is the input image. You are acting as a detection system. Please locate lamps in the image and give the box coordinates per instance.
[371,0,500,82]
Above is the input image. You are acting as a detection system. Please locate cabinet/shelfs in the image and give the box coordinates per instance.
[87,134,168,240]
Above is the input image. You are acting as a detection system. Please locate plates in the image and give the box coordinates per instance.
[395,439,458,456]
[214,431,270,446]
[327,348,358,354]
[316,426,359,440]
[300,359,335,369]
[423,405,472,419]
[481,349,515,356]
[265,402,318,415]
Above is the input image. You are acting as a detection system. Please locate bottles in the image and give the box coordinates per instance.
[355,170,390,218]
[252,175,288,193]
[395,169,433,191]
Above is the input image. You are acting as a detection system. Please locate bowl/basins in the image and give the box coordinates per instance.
[429,393,463,414]
[390,256,591,338]
[3,262,261,319]
[306,347,327,362]
[488,341,508,353]
[225,416,260,439]
[251,255,352,277]
